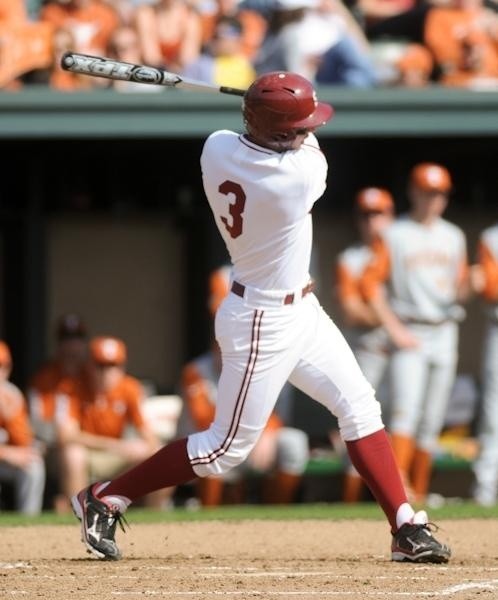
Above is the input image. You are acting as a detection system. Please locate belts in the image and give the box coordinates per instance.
[231,281,315,304]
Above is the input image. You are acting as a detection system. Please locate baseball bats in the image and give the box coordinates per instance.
[61,50,247,97]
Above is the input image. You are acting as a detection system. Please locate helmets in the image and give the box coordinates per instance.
[242,71,334,130]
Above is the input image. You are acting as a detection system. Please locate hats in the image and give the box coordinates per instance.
[360,163,451,212]
[90,337,127,362]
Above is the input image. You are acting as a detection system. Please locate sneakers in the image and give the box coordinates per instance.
[391,510,450,562]
[70,482,120,559]
